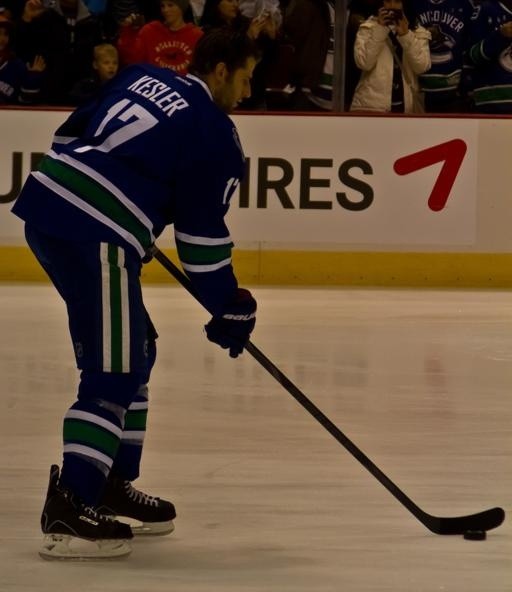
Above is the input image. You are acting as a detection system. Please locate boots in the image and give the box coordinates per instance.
[95,472,179,522]
[39,462,134,540]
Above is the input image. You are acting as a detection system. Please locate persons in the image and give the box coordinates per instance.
[10,26,257,539]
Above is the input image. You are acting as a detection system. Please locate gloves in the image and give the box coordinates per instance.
[201,287,258,359]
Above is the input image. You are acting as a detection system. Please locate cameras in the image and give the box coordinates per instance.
[382,9,402,21]
[257,8,271,23]
[43,0,60,9]
[132,15,145,26]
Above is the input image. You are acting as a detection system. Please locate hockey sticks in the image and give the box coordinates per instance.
[148,244,505,536]
